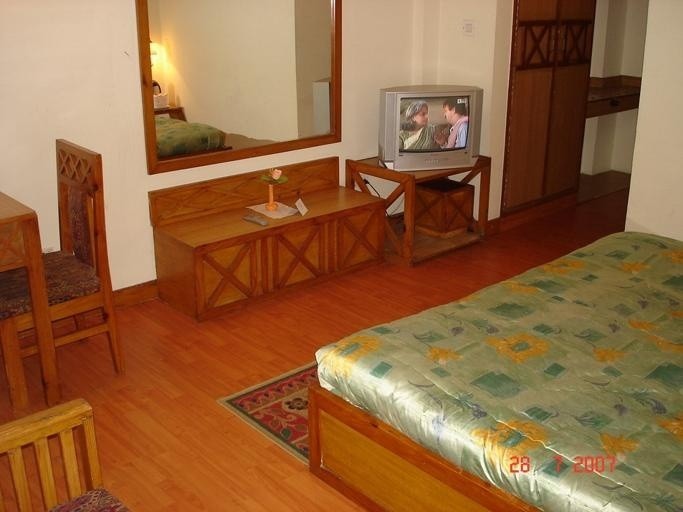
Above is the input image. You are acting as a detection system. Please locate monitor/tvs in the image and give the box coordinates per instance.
[378,85,484,172]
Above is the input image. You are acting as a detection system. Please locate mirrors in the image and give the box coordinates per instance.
[134,0,343,176]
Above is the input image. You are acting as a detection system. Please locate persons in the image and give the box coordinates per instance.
[398,100,437,151]
[433,97,467,149]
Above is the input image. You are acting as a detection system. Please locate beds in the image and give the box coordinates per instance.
[308,231,683,512]
[154,115,231,162]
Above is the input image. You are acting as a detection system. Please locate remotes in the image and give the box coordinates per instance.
[243,216,267,226]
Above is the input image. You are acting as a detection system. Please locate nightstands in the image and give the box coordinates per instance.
[155,107,187,122]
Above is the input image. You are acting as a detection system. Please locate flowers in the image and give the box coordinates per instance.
[259,168,289,186]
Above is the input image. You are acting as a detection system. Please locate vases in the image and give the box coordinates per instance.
[265,184,279,212]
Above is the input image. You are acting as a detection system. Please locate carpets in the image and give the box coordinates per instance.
[219,362,314,469]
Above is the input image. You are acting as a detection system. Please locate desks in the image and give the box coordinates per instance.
[0,191,62,408]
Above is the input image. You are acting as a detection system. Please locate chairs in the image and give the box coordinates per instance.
[0,400,137,512]
[0,141,124,410]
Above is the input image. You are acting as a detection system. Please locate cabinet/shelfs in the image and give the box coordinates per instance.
[414,178,475,239]
[148,154,386,323]
[344,153,490,268]
[498,0,596,214]
